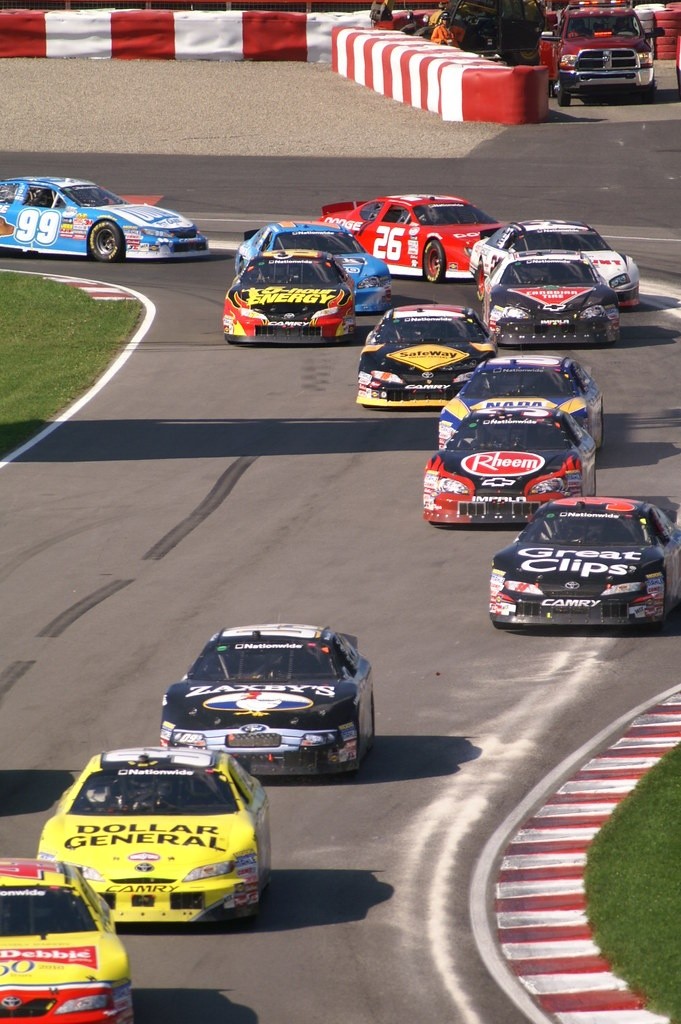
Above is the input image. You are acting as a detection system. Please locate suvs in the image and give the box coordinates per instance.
[542,1,664,106]
[400,0,547,66]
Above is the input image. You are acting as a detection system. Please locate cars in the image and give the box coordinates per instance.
[317,194,507,285]
[488,497,681,631]
[160,624,376,777]
[439,355,605,449]
[422,407,596,526]
[356,304,499,409]
[0,857,134,1023]
[468,218,640,308]
[38,747,273,923]
[223,250,354,346]
[235,221,393,311]
[483,249,620,348]
[0,176,209,262]
